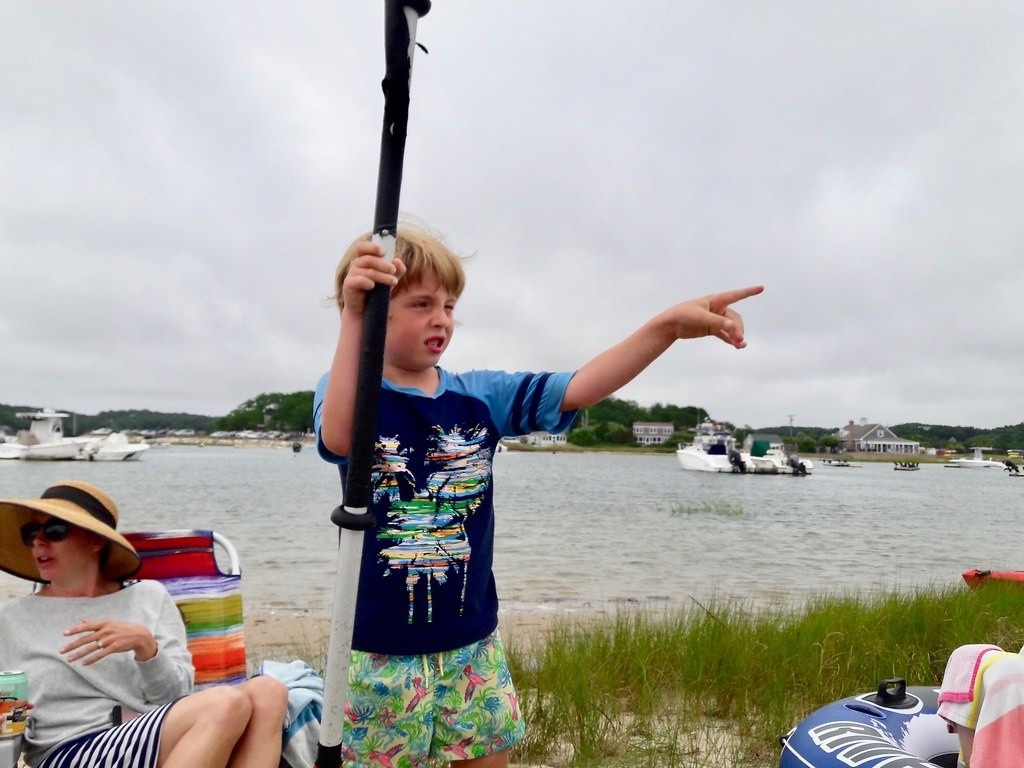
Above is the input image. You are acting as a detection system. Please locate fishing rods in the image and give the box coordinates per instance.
[314,0,432,768]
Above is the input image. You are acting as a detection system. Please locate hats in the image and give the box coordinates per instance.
[0,480,142,584]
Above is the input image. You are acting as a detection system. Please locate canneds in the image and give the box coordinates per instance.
[0,670,28,738]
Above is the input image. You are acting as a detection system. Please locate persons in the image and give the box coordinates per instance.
[0,479,289,768]
[313,212,764,768]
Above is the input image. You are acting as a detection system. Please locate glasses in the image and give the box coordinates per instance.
[20,516,71,546]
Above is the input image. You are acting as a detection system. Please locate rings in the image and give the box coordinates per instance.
[96,639,103,649]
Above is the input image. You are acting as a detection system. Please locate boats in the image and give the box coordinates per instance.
[1008,472,1024,477]
[944,447,1008,470]
[893,460,921,471]
[822,458,862,468]
[675,417,813,477]
[961,569,1024,590]
[0,408,150,461]
